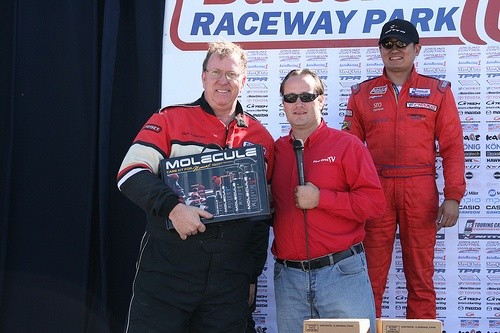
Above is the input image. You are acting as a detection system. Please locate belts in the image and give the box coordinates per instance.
[276,243,363,269]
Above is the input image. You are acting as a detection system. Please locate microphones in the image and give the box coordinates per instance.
[293,139,306,213]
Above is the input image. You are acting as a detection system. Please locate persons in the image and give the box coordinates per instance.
[270,68,387,333]
[118,41,274,333]
[342,18,467,323]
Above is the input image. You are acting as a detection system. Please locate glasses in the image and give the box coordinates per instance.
[381,40,412,49]
[206,68,243,80]
[282,92,319,102]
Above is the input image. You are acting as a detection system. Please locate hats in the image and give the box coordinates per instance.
[378,18,419,45]
